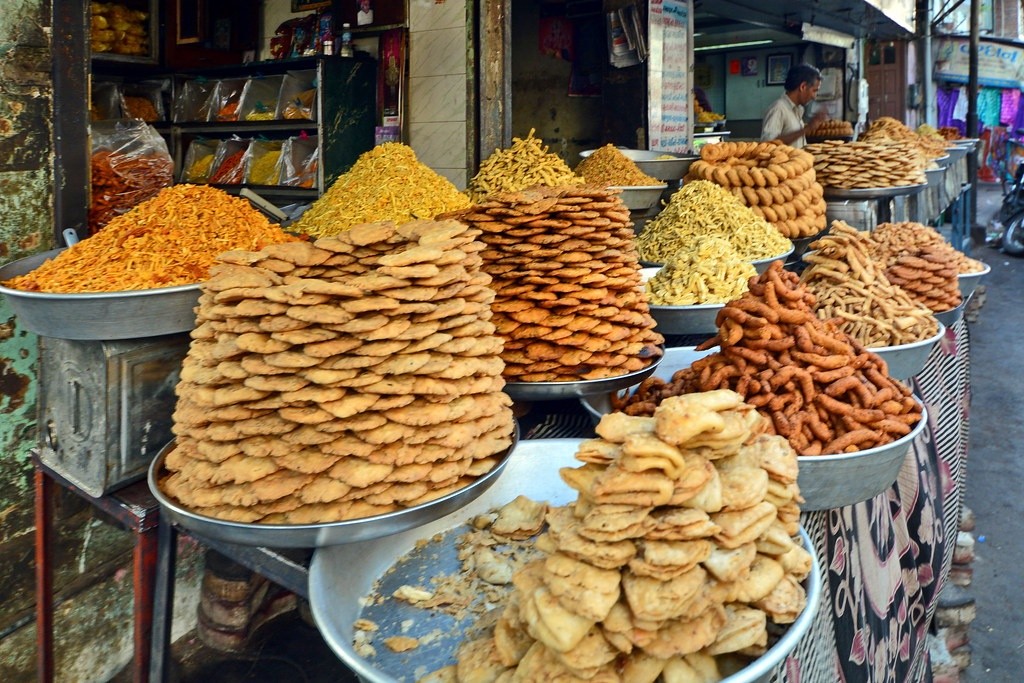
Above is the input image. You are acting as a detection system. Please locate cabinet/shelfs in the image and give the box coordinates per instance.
[87,0,411,215]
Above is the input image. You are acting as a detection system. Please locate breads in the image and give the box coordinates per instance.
[806,120,854,135]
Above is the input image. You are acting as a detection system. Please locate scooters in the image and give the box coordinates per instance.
[983,129,1024,258]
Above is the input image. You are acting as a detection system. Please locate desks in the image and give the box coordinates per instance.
[29,446,159,683]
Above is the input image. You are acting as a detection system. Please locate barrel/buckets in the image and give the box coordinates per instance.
[826,200,868,233]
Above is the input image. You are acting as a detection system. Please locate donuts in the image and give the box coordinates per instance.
[685,141,827,239]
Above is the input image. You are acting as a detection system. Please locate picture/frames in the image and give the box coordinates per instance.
[766,53,793,85]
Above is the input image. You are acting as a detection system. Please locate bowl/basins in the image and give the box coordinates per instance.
[1,143,993,683]
[696,118,728,127]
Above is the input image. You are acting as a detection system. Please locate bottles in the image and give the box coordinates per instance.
[374,107,400,144]
[322,32,353,56]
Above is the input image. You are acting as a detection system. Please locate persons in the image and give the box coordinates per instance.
[759,62,830,150]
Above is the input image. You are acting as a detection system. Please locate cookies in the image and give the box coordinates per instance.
[160,217,515,525]
[435,183,664,382]
[884,245,962,312]
[802,139,929,189]
